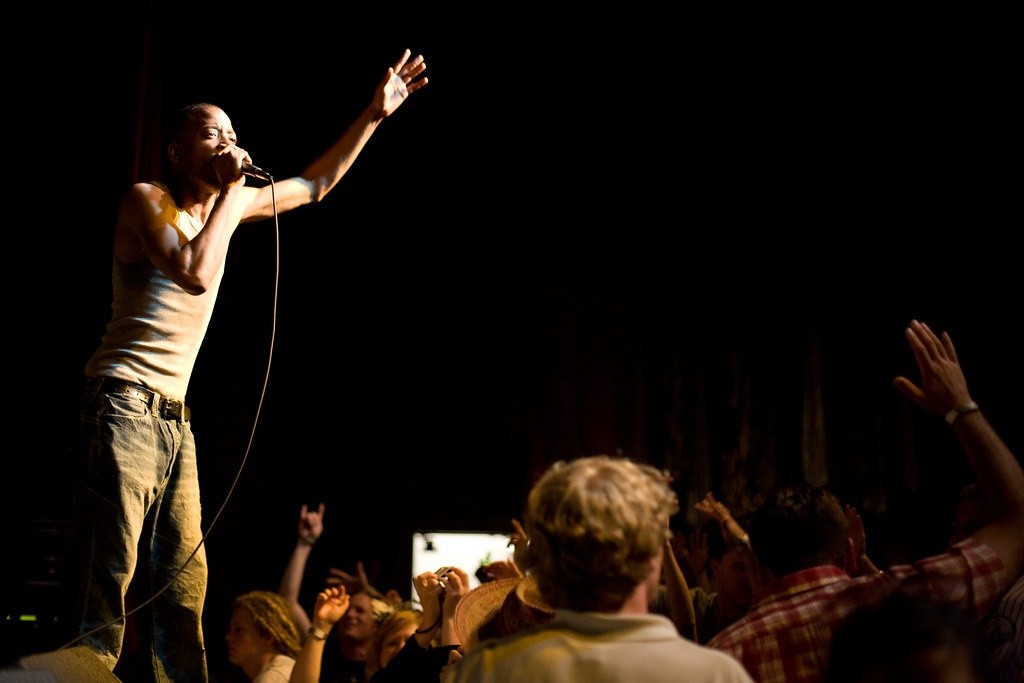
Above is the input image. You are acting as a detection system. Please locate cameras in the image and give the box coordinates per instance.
[433,567,455,598]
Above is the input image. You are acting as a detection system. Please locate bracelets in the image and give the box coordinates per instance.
[415,618,440,634]
[944,400,980,425]
[310,623,330,640]
[297,535,315,545]
[742,533,749,544]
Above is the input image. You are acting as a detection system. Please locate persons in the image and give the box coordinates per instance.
[224,319,1024,683]
[78,48,430,683]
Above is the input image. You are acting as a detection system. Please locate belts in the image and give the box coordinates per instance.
[89,377,191,421]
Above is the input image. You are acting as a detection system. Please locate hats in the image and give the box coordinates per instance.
[453,575,556,656]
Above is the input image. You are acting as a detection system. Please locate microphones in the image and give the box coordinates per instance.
[241,159,273,181]
